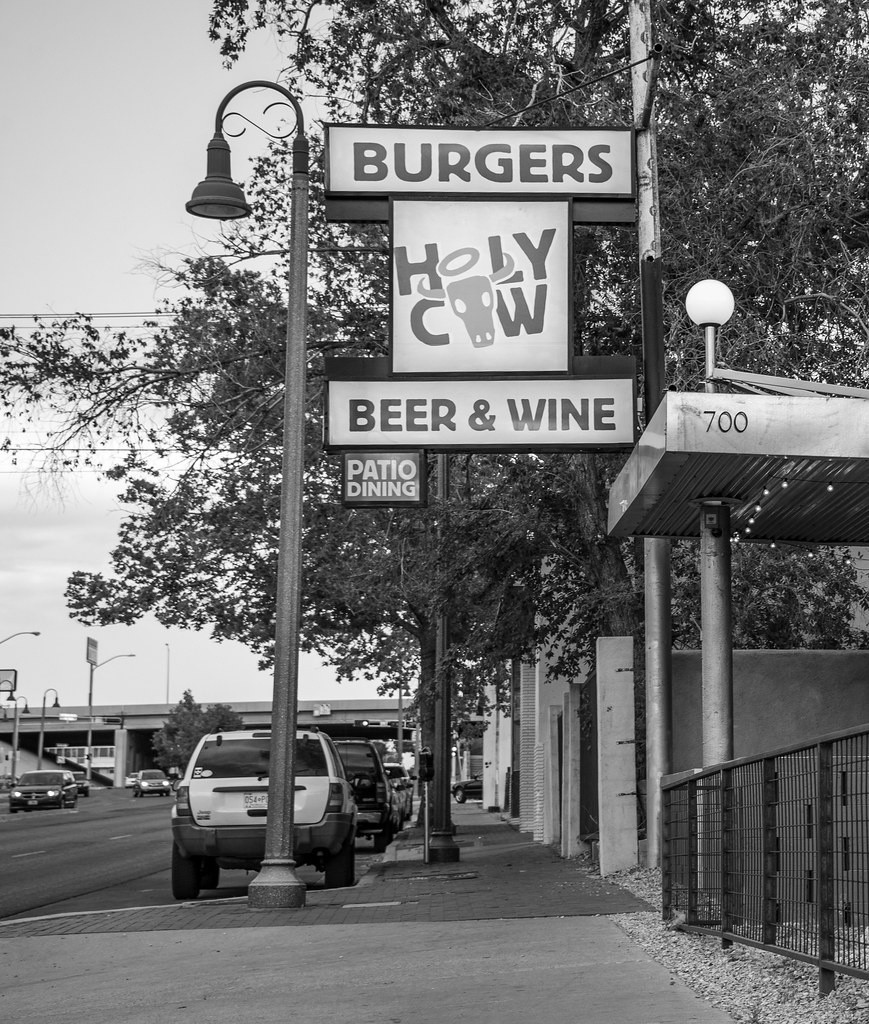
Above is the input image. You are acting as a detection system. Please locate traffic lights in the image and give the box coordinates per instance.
[353,720,369,727]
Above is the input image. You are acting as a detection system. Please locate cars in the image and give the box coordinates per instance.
[384,770,409,834]
[449,770,484,803]
[0,773,19,789]
[9,770,78,811]
[126,772,137,788]
[74,771,90,797]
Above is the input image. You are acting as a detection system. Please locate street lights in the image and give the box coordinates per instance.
[37,688,62,770]
[685,277,737,913]
[184,75,311,909]
[9,696,32,787]
[84,653,135,797]
[164,642,169,704]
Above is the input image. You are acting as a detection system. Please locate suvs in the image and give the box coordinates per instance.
[331,740,396,854]
[133,768,171,798]
[384,762,418,820]
[171,722,373,902]
